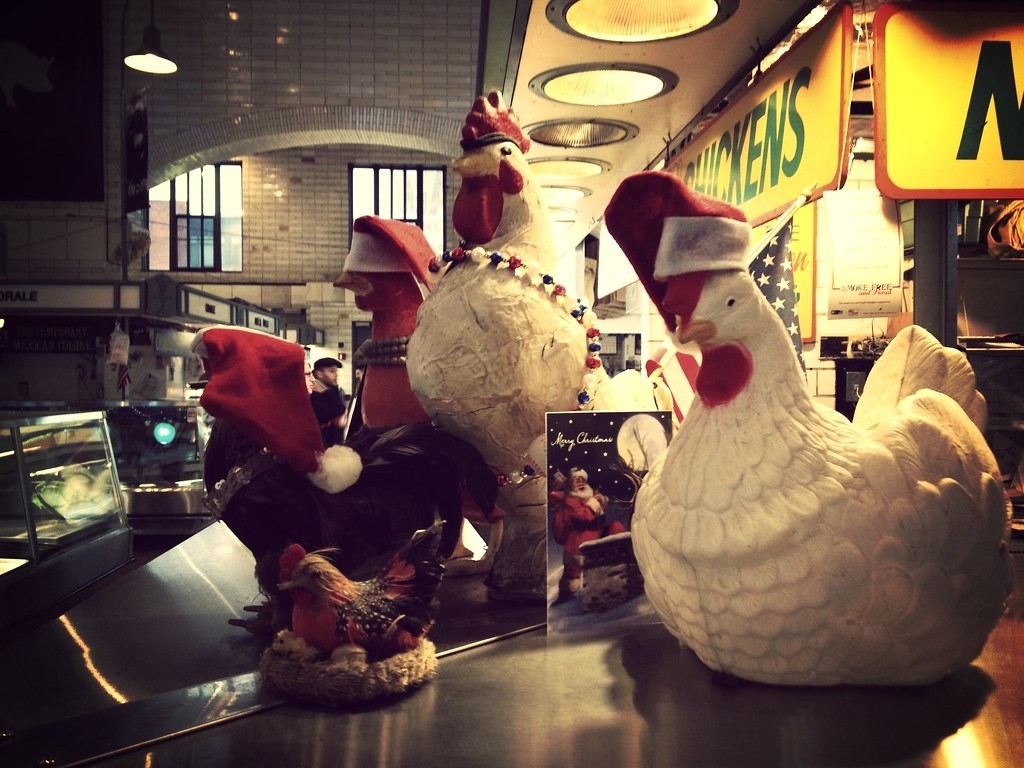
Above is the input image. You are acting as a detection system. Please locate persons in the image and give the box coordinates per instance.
[304,357,365,451]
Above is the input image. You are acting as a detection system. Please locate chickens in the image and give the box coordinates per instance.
[633,263,1015,684]
[186,354,499,632]
[333,270,504,564]
[276,520,447,652]
[408,89,651,578]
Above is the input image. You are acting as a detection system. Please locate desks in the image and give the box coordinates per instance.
[0,519,1024,768]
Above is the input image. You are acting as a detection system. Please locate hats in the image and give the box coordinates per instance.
[315,358,342,368]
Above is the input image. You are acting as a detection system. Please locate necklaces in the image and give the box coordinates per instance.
[131,406,163,425]
[359,335,410,365]
[426,246,602,484]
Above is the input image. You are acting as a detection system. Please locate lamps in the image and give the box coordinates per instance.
[123,0,179,76]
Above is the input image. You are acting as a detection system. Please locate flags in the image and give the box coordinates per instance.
[651,217,809,450]
